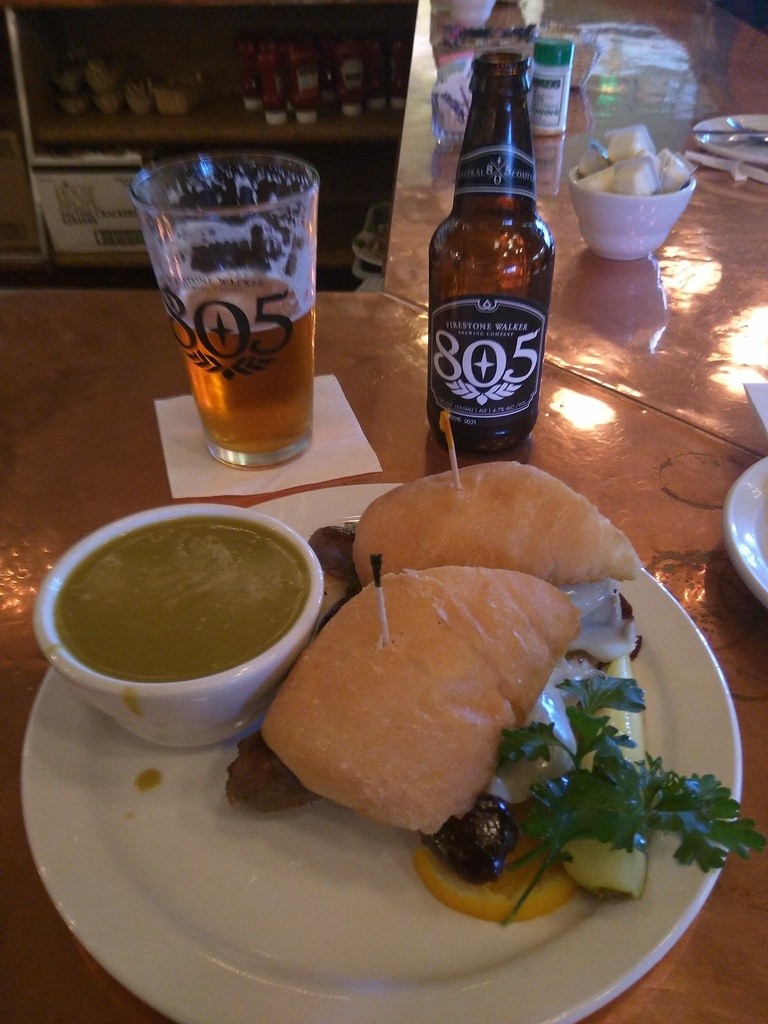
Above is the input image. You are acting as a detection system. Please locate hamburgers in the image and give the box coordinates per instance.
[224,565,583,839]
[307,457,648,663]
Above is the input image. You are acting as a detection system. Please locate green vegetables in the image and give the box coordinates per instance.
[485,669,764,922]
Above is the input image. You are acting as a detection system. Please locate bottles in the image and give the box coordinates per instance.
[426,51,555,456]
[526,37,576,135]
[236,28,412,126]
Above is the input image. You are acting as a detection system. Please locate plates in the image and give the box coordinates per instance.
[21,483,742,1024]
[691,113,768,164]
[722,455,768,612]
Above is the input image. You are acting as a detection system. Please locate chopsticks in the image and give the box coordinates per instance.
[693,129,767,135]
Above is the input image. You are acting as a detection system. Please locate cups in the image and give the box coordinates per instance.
[429,0,542,151]
[128,148,321,470]
[50,50,209,115]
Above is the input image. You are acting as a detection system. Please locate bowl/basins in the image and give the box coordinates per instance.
[568,166,698,261]
[32,502,324,748]
